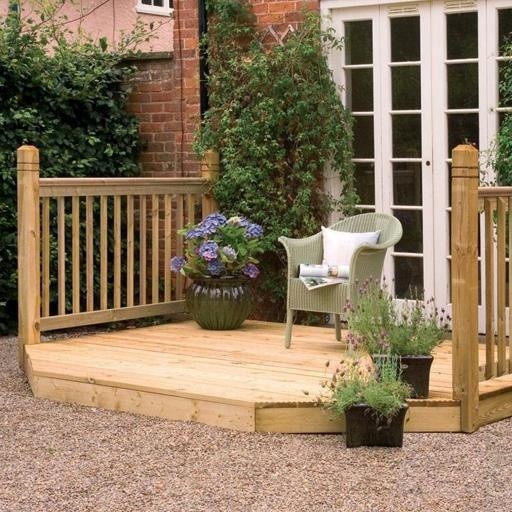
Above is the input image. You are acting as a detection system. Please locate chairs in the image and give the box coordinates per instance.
[277,212,404,349]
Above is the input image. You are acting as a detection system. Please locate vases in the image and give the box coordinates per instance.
[185,277,250,329]
[344,401,409,449]
[369,356,434,398]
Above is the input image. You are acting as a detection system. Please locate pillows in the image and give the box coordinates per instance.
[321,225,380,280]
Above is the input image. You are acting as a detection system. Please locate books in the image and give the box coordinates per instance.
[298,263,350,291]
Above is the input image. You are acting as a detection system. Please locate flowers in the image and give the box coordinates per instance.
[340,274,452,355]
[169,214,263,282]
[302,356,416,426]
